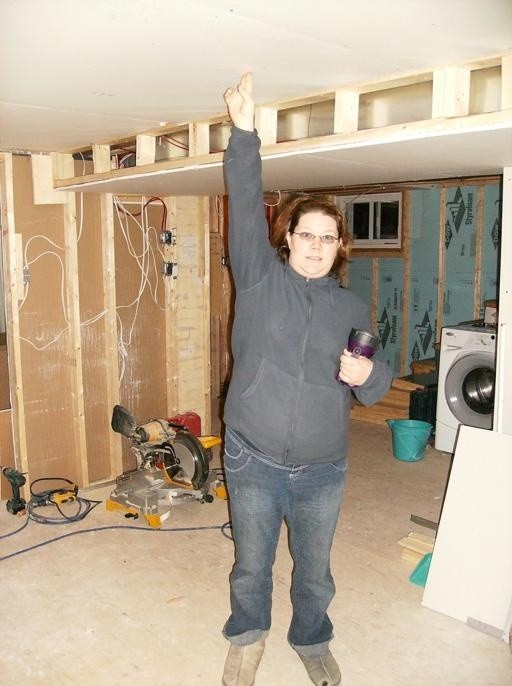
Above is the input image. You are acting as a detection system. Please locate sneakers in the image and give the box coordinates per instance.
[288,641,341,686]
[221,635,268,686]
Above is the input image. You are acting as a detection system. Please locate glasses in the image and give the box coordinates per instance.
[293,232,340,244]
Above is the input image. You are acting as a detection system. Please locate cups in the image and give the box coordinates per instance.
[337,327,382,388]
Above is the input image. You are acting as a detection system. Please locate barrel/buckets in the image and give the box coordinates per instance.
[385,419,433,462]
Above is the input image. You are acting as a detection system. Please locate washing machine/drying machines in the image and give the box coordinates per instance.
[434,323,497,453]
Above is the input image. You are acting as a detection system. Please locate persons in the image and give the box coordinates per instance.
[218,72,396,685]
